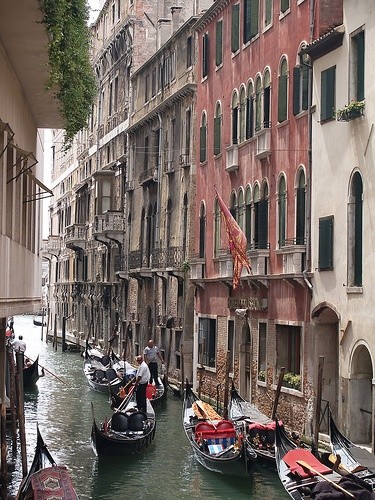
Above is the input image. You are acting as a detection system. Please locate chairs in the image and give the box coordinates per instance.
[93,367,117,383]
[111,412,148,433]
[196,421,236,449]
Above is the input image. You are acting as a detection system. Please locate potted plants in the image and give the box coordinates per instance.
[278,370,302,391]
[257,370,266,382]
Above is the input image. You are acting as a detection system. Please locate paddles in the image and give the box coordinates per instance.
[197,400,217,430]
[296,460,355,498]
[105,375,136,432]
[13,347,71,389]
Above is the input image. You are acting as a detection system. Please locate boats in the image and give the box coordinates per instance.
[80,338,127,395]
[313,400,375,493]
[15,422,80,500]
[228,377,312,474]
[14,354,40,388]
[110,347,165,409]
[273,417,375,500]
[182,376,259,476]
[89,393,156,455]
[6,319,15,345]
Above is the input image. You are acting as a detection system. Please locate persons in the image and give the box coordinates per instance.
[133,354,152,413]
[10,334,26,371]
[142,339,165,386]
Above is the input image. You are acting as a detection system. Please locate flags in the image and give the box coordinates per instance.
[212,186,253,293]
